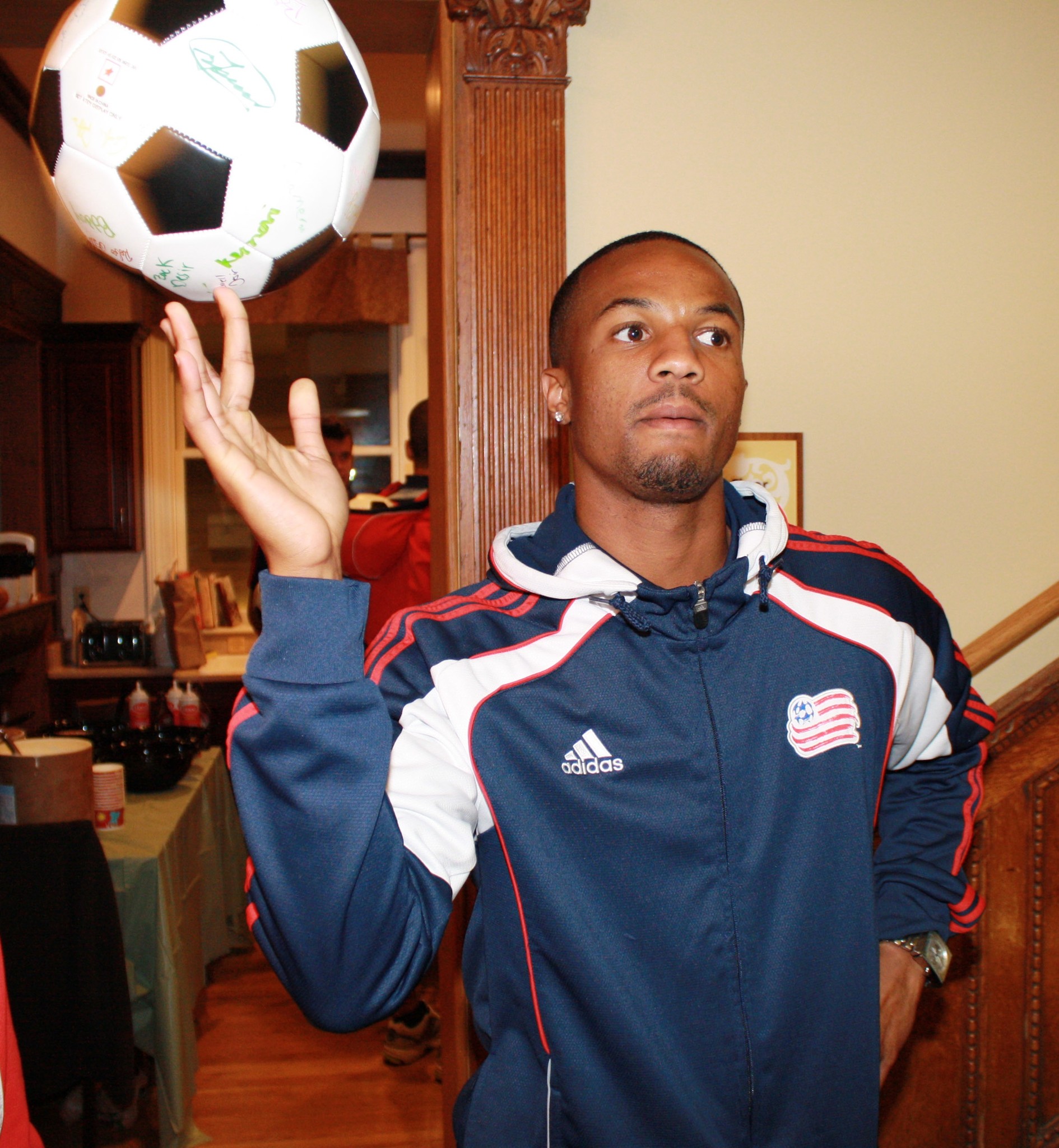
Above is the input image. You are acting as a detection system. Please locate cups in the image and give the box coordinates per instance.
[92,762,126,830]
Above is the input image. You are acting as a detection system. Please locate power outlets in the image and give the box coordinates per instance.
[72,585,90,612]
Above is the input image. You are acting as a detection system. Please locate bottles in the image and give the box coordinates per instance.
[127,680,149,728]
[165,679,183,727]
[180,681,201,729]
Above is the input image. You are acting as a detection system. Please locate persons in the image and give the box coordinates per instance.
[343,395,444,1088]
[245,417,357,638]
[160,231,1000,1148]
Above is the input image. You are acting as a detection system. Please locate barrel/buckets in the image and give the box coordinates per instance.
[0,736,92,825]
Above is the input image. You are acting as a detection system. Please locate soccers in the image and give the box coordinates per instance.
[22,0,380,302]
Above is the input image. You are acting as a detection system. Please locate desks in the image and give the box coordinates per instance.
[98,743,255,1142]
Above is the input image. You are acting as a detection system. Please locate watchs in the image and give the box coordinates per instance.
[892,927,955,992]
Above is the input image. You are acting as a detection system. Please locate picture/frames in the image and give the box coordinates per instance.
[722,432,804,530]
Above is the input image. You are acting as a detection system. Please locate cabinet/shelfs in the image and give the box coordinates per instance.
[57,319,154,556]
